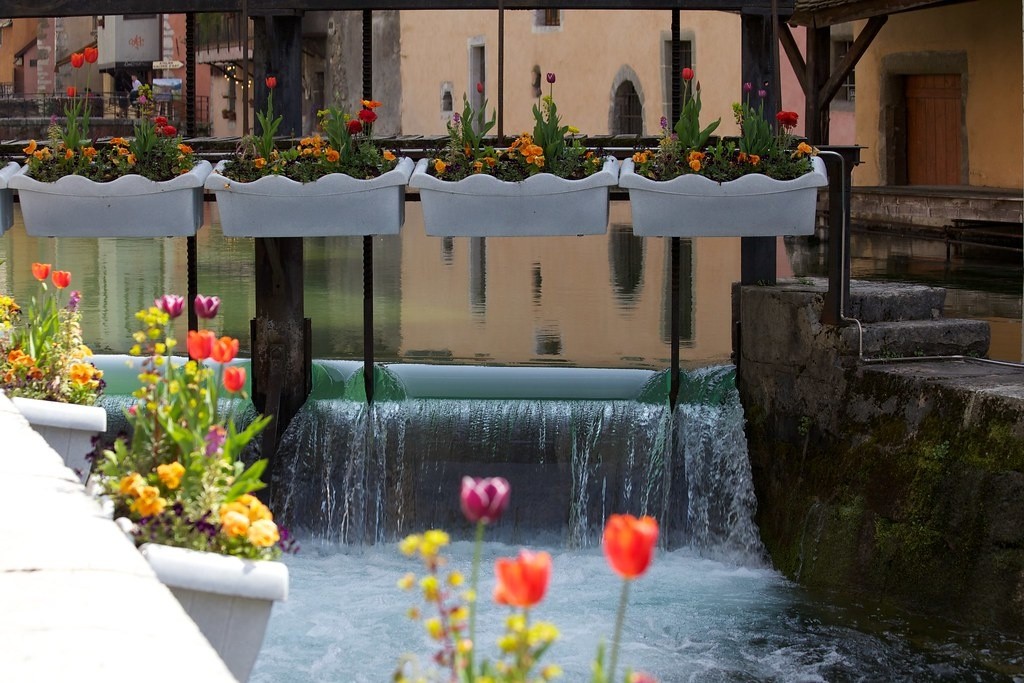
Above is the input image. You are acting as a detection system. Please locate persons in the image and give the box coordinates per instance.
[81,87,103,117]
[129,71,143,92]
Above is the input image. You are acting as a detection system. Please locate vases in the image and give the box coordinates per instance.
[11,396,107,485]
[0,161,23,236]
[92,476,291,682]
[204,157,415,238]
[618,157,828,237]
[408,155,620,237]
[8,160,213,238]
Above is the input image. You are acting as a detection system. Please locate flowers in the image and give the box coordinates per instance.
[632,68,821,182]
[379,476,666,683]
[0,262,106,407]
[24,48,197,182]
[429,72,605,182]
[223,76,398,182]
[84,292,300,561]
